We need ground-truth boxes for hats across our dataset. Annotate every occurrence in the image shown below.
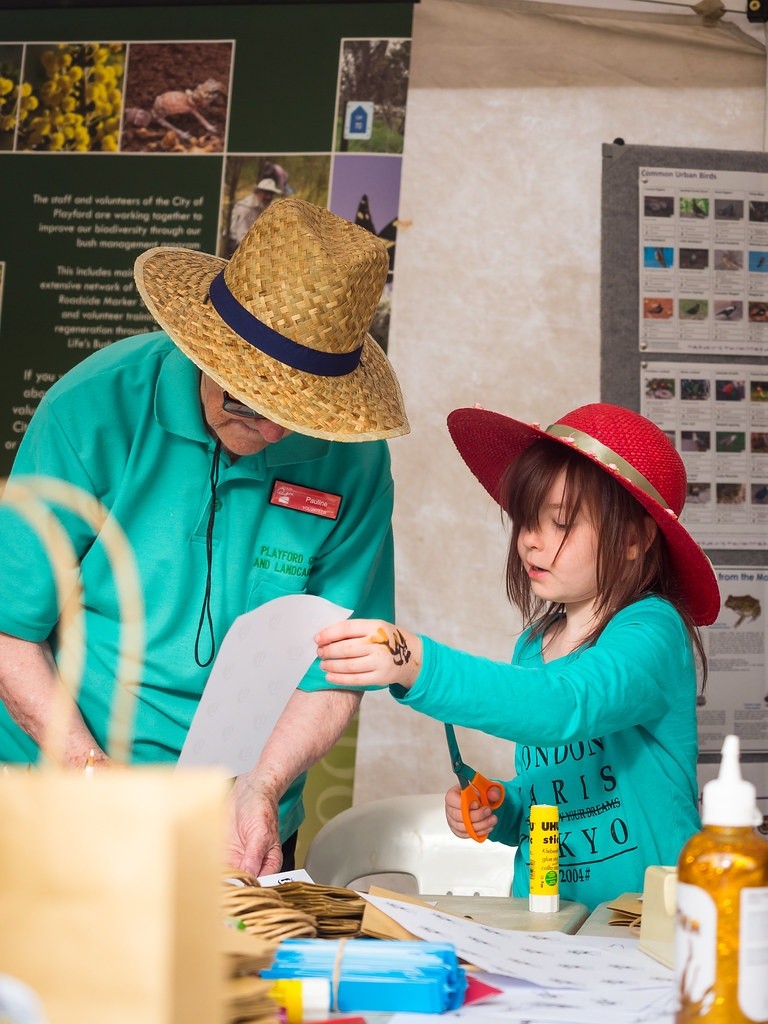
[134,198,411,443]
[447,402,721,627]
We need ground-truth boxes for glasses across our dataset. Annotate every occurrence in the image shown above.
[222,389,267,419]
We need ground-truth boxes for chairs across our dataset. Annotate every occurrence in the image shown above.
[307,793,516,896]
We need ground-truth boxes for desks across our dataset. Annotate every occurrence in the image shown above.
[423,893,640,938]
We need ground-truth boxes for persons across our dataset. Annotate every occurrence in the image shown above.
[315,403,722,913]
[222,177,281,260]
[0,197,411,878]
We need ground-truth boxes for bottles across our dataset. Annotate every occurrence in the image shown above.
[675,736,768,1024]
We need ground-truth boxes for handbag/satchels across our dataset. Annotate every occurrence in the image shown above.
[0,474,235,1024]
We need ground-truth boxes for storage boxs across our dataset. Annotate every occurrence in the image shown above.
[261,936,469,1014]
[637,864,676,971]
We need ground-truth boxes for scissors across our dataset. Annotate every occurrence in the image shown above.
[444,723,505,844]
